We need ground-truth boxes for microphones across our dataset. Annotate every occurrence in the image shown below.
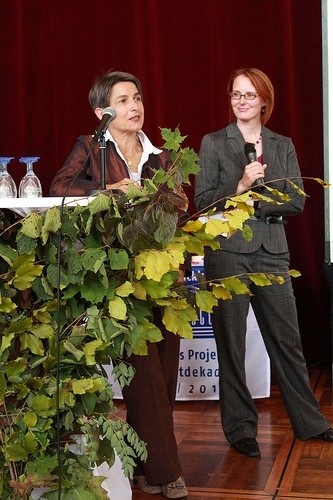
[91,107,116,143]
[244,143,265,191]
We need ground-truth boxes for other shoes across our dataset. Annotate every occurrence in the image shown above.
[130,475,163,495]
[162,474,188,498]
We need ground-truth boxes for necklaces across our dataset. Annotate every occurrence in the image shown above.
[253,133,262,145]
[122,147,139,168]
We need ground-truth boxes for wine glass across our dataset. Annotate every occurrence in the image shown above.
[0,157,17,199]
[18,157,42,198]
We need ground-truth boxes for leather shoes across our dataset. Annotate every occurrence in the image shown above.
[230,438,261,457]
[313,426,333,442]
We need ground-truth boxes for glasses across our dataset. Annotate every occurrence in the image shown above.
[228,91,260,100]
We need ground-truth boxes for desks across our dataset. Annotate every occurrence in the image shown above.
[98,257,271,400]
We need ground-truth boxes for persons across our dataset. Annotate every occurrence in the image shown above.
[193,68,333,455]
[50,71,193,498]
